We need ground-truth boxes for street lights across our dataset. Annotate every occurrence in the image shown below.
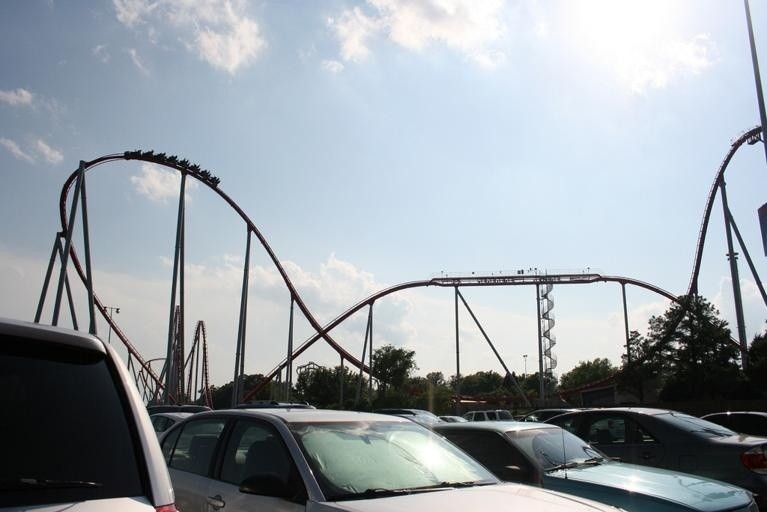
[103,306,120,345]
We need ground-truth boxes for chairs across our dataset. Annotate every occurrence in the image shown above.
[241,440,290,490]
[596,429,619,443]
[187,435,222,477]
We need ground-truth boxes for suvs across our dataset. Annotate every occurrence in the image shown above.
[462,409,514,421]
[259,400,316,409]
[0,316,178,512]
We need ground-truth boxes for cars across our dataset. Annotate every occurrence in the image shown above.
[539,407,767,494]
[700,412,767,437]
[518,408,579,423]
[156,407,627,512]
[146,403,226,446]
[374,408,442,427]
[439,414,469,422]
[426,420,761,512]
